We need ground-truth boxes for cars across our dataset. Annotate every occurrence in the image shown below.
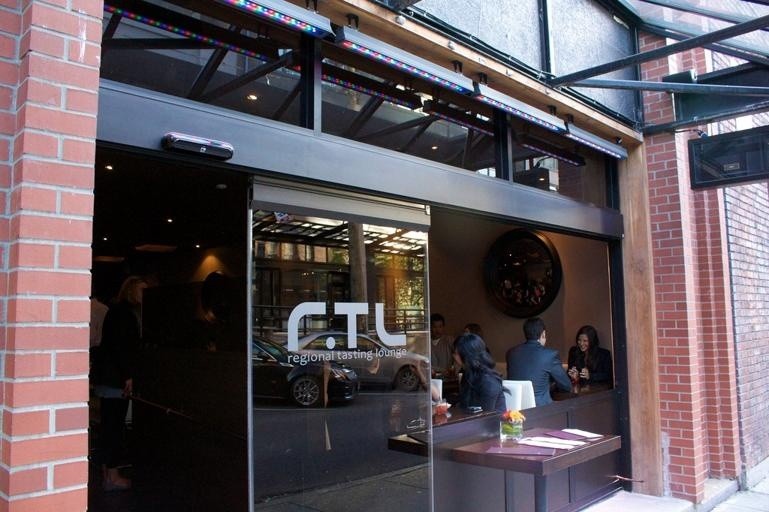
[282,330,431,392]
[252,335,361,407]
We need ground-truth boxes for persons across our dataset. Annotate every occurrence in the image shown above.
[408,358,441,402]
[464,323,491,355]
[505,317,573,407]
[430,313,456,378]
[451,333,507,413]
[93,274,152,493]
[566,325,612,384]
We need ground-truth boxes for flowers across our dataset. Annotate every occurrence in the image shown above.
[500,409,527,436]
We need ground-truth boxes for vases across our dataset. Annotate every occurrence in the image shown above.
[500,423,522,441]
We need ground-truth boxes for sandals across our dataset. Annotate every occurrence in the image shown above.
[104,477,132,491]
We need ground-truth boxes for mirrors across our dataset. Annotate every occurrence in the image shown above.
[482,229,563,317]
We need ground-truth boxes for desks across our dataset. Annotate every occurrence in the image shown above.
[435,428,623,510]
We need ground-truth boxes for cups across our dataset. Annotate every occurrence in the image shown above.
[431,379,443,405]
[499,419,523,442]
